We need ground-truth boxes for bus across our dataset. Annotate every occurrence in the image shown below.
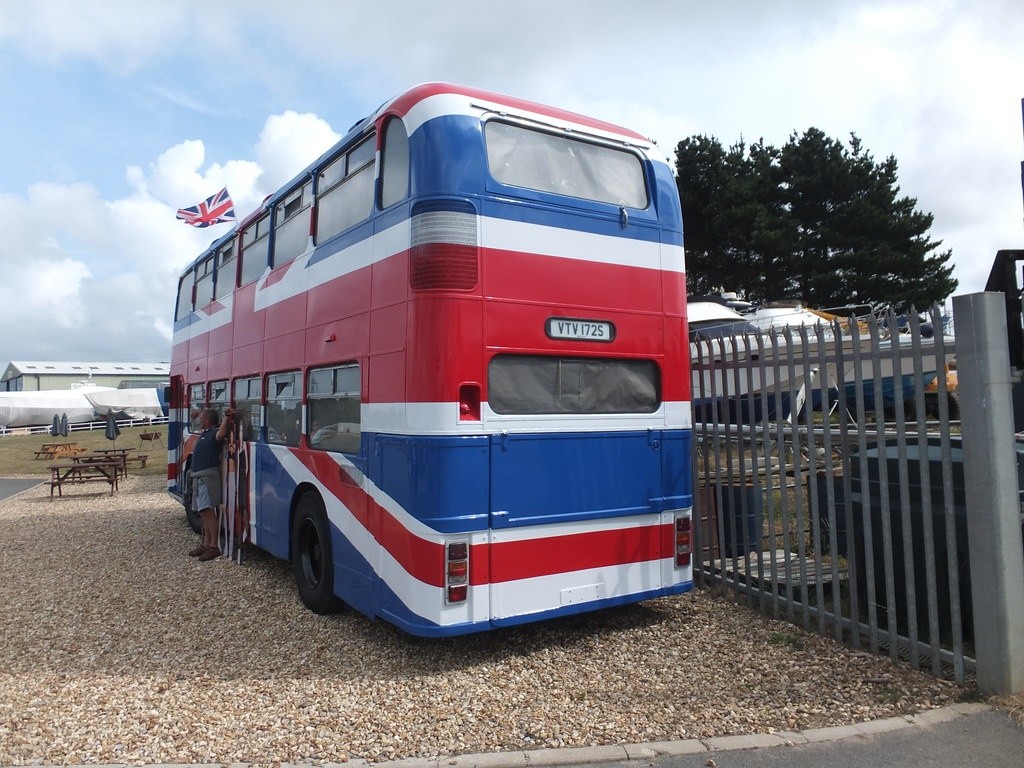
[156,80,696,639]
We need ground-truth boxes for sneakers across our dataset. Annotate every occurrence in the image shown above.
[199,546,221,561]
[189,545,208,556]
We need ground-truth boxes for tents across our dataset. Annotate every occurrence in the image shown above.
[0,387,170,427]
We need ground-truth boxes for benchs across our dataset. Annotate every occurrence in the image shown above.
[44,448,87,456]
[32,450,74,459]
[54,473,124,492]
[67,458,141,481]
[43,478,120,498]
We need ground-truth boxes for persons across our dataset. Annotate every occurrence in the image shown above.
[189,407,233,561]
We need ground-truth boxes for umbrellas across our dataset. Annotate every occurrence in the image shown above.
[52,413,68,443]
[105,408,121,458]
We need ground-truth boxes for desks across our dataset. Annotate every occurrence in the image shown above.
[46,462,122,502]
[35,442,78,459]
[68,453,129,479]
[93,448,137,478]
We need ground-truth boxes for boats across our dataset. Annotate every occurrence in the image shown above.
[684,288,957,421]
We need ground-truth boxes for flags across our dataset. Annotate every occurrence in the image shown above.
[176,186,237,228]
